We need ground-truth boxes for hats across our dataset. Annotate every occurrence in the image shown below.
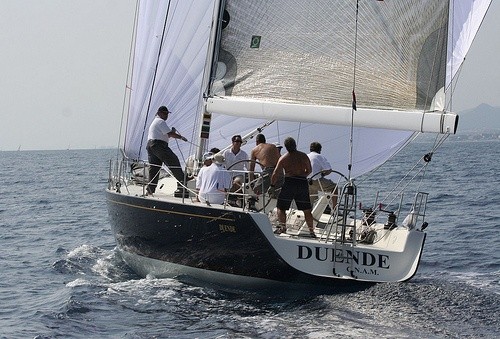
[155,106,171,116]
[274,142,283,147]
[203,152,213,160]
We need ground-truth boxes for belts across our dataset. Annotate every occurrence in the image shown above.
[149,139,166,143]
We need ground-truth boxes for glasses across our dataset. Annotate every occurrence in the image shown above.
[233,140,243,143]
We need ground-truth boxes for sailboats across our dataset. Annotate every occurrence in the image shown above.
[103,1,493,298]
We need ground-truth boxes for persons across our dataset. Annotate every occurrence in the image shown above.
[196,135,338,238]
[146,105,194,196]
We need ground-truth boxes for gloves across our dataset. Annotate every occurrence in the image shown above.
[181,136,187,141]
[172,127,176,133]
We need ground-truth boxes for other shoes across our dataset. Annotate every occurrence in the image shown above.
[310,233,316,237]
[274,228,286,233]
[146,187,153,193]
[184,177,194,181]
[330,210,342,215]
